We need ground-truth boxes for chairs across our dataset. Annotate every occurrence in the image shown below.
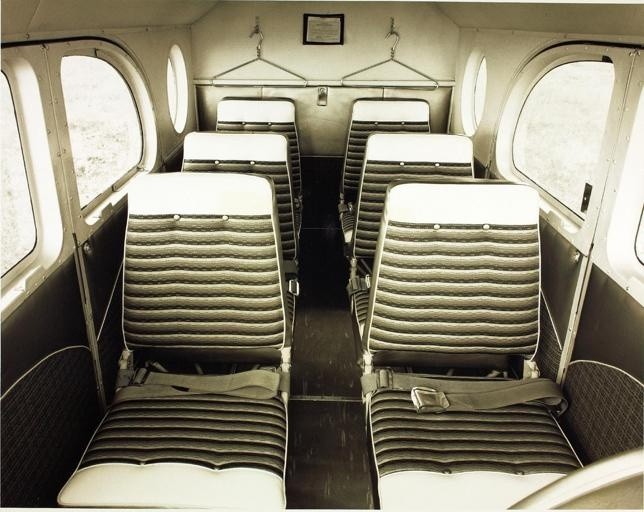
[348,132,475,368]
[215,98,304,235]
[361,180,584,510]
[338,98,431,255]
[56,173,289,509]
[182,131,299,338]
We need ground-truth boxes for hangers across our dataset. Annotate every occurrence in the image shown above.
[340,32,439,92]
[210,31,308,91]
